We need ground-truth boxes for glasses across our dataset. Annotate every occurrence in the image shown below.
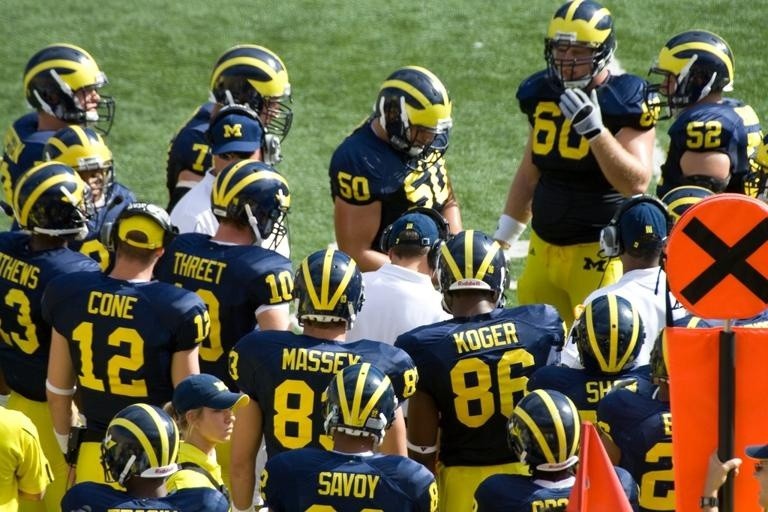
[754,463,768,472]
[216,151,255,160]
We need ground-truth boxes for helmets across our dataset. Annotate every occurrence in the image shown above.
[749,133,768,204]
[650,315,712,386]
[209,160,291,240]
[42,125,115,208]
[324,363,400,446]
[292,249,365,329]
[209,44,293,143]
[23,43,109,126]
[11,161,92,241]
[508,389,581,473]
[100,403,180,487]
[544,0,618,94]
[376,65,453,173]
[649,29,733,107]
[571,295,646,374]
[436,229,510,314]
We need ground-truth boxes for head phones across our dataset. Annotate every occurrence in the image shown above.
[209,104,285,167]
[597,193,676,259]
[380,207,450,269]
[100,201,179,252]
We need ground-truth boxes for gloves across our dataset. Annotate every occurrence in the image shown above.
[559,88,605,143]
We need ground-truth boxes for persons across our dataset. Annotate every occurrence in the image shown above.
[1,160,103,511]
[227,250,418,512]
[492,0,660,331]
[645,30,768,200]
[472,389,640,512]
[343,212,453,345]
[1,374,250,511]
[328,66,461,273]
[525,196,768,512]
[394,230,568,512]
[171,104,292,258]
[260,363,438,512]
[166,44,294,211]
[0,43,134,273]
[41,202,211,489]
[156,160,295,392]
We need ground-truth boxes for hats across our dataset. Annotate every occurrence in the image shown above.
[387,213,439,246]
[172,373,251,418]
[745,444,768,460]
[119,213,165,250]
[618,201,667,249]
[209,111,263,154]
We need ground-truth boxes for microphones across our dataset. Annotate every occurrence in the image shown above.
[96,195,124,242]
